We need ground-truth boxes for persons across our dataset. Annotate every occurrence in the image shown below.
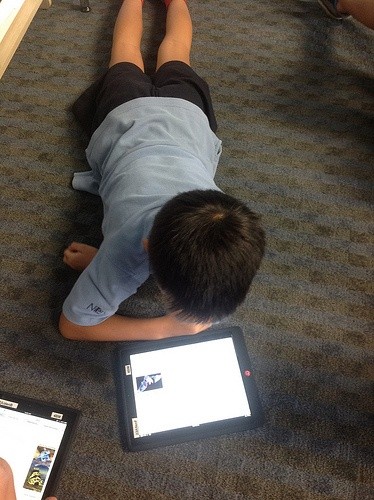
[0,456,58,500]
[318,0,374,31]
[58,0,267,341]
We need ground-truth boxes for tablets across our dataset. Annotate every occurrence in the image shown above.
[114,326,265,453]
[0,391,82,500]
